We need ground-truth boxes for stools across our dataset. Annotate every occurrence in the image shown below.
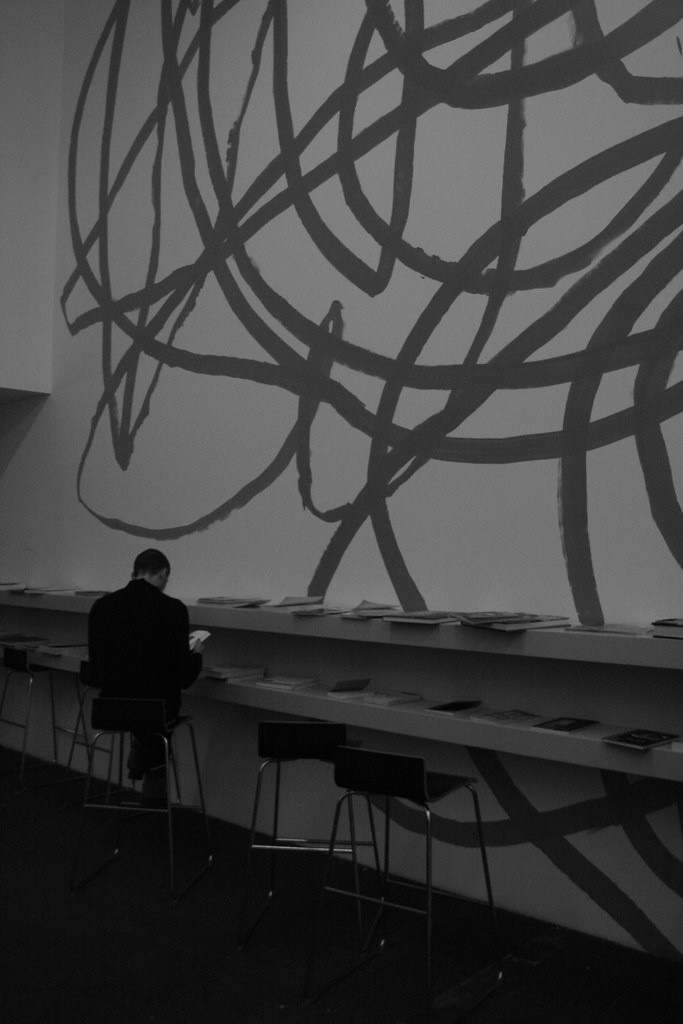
[70,699,211,900]
[301,744,506,1024]
[0,643,93,788]
[232,719,389,950]
[67,658,134,807]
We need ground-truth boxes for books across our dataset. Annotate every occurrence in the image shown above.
[0,583,112,648]
[188,594,682,750]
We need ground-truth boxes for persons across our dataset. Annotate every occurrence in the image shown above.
[88,548,204,780]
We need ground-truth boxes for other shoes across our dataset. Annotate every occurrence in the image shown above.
[128,753,154,781]
